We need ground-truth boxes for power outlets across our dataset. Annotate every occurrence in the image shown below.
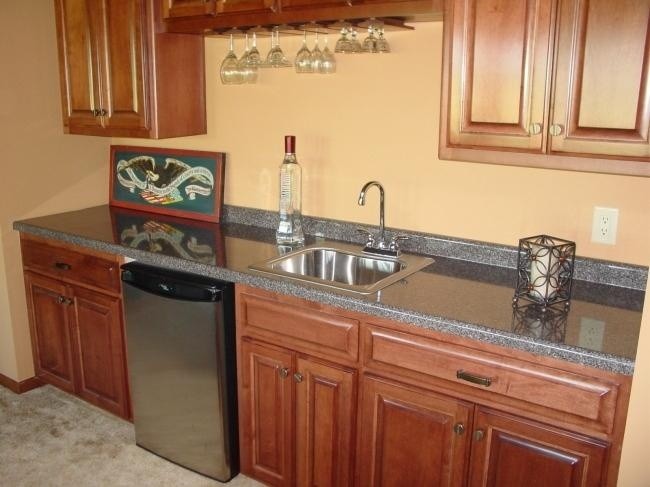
[588,206,618,246]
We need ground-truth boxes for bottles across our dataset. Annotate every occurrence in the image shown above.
[275,134,304,248]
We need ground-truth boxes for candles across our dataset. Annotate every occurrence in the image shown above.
[529,243,561,302]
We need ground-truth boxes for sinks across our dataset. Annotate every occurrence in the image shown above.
[261,247,405,291]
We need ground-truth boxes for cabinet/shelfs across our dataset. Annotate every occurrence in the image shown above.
[16,229,132,426]
[357,315,635,486]
[436,0,650,179]
[234,281,364,486]
[153,0,447,33]
[55,0,207,138]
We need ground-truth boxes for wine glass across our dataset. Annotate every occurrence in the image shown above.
[220,17,391,86]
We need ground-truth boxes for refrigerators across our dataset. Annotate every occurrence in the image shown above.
[119,261,236,484]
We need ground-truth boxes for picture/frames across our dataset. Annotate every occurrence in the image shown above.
[107,144,226,225]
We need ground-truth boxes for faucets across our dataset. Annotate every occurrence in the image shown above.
[358,181,389,250]
